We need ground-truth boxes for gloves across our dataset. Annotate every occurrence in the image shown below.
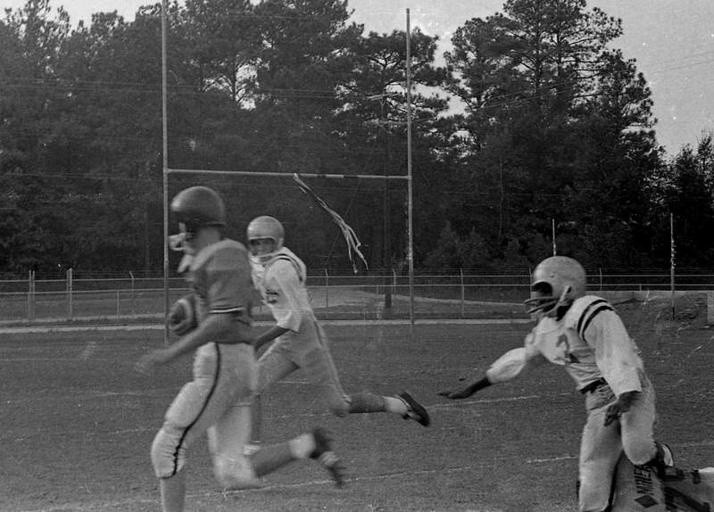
[436,390,469,399]
[604,393,632,426]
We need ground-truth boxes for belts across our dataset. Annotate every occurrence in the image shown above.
[581,377,607,395]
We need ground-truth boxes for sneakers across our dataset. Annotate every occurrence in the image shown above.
[309,428,343,489]
[392,391,429,426]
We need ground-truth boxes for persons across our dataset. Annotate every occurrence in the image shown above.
[436,256,674,511]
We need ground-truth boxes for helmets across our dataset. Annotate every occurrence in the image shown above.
[529,255,586,300]
[247,215,284,254]
[170,186,225,225]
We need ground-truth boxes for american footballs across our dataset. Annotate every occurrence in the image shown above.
[166,293,196,336]
